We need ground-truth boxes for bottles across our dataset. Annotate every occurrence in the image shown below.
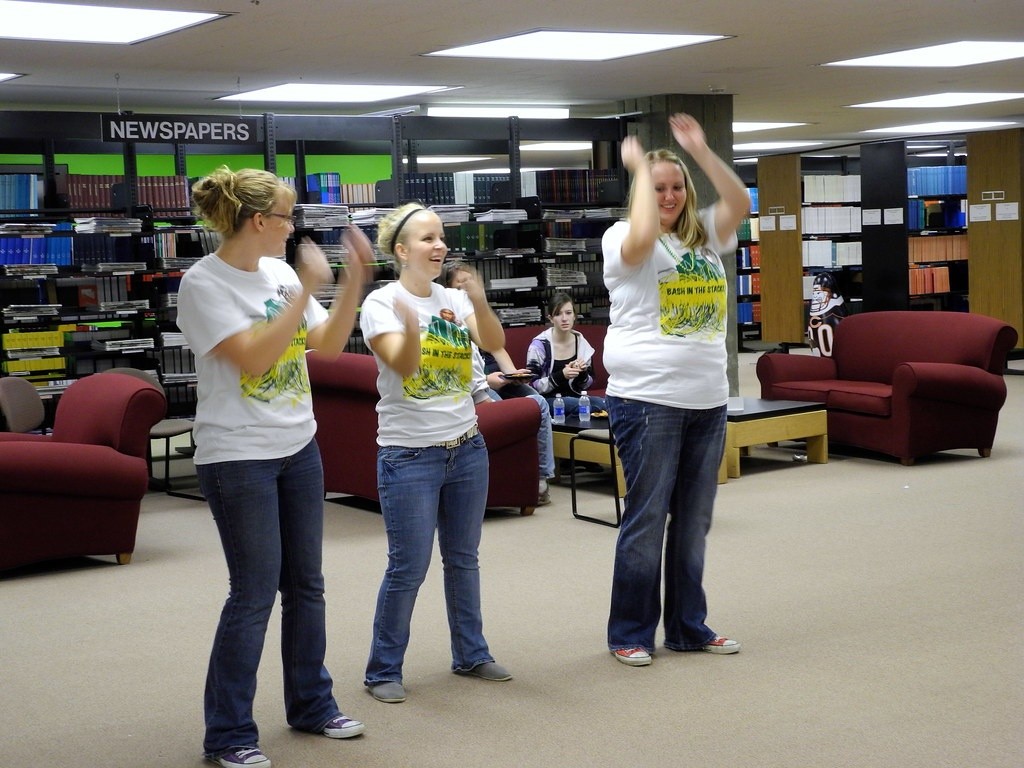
[553,394,565,424]
[578,391,591,421]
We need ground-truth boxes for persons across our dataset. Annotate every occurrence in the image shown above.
[438,261,555,505]
[527,292,606,470]
[603,115,751,667]
[175,168,372,768]
[360,204,512,702]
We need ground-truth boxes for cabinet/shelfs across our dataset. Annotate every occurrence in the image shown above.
[0,113,631,411]
[861,139,968,314]
[761,153,863,351]
[736,165,764,344]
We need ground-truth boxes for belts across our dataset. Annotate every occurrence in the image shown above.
[430,424,478,450]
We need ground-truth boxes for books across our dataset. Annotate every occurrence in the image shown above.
[908,166,969,295]
[801,174,862,300]
[737,217,760,323]
[282,168,625,354]
[0,170,223,403]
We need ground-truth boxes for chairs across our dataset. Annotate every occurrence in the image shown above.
[0,376,44,432]
[109,368,203,492]
[570,421,622,531]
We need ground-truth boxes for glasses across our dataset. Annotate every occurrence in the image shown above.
[250,211,296,225]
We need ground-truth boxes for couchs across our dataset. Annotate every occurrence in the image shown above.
[491,324,616,404]
[303,347,539,514]
[755,311,1019,465]
[0,374,166,565]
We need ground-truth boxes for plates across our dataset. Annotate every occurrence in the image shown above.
[591,416,608,420]
[504,373,539,378]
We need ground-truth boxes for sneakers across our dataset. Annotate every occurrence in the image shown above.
[611,646,652,666]
[323,714,365,739]
[702,636,741,654]
[204,748,271,768]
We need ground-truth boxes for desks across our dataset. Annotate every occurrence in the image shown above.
[722,397,828,478]
[549,413,728,496]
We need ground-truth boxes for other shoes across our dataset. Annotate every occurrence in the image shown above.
[367,681,407,703]
[537,481,551,506]
[452,661,513,681]
[559,464,572,476]
[585,462,604,473]
